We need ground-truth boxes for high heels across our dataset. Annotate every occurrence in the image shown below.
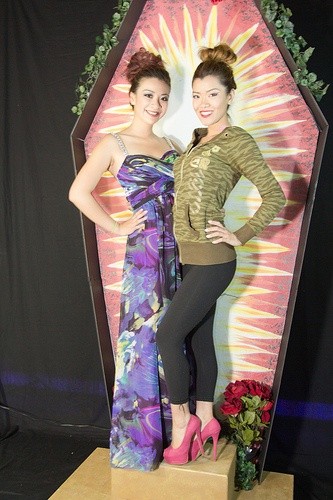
[188,418,221,461]
[163,413,205,464]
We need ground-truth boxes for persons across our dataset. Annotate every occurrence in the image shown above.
[67,47,186,472]
[154,41,287,467]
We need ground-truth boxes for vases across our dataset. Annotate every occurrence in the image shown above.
[236,444,259,489]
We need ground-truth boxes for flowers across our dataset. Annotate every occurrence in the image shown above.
[220,380,273,490]
